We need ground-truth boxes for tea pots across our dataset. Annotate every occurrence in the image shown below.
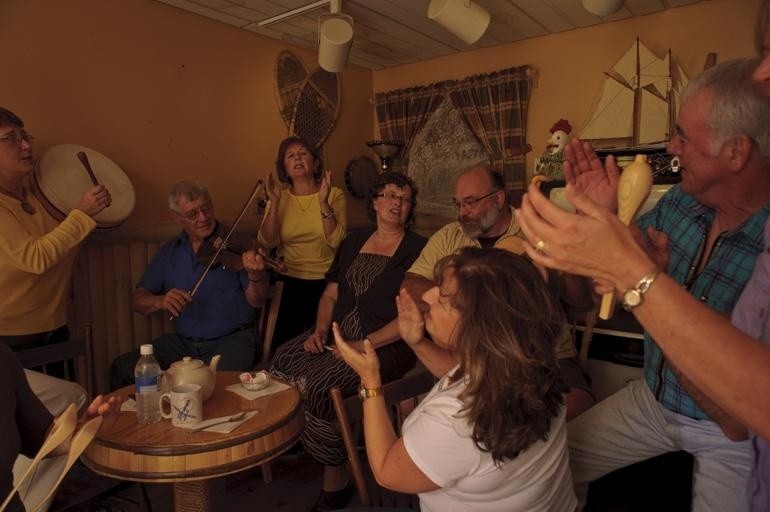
[157,353,221,402]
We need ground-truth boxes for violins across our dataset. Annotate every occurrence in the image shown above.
[189,220,289,281]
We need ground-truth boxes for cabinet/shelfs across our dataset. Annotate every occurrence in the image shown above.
[574,324,643,404]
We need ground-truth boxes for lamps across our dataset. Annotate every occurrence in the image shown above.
[367,140,405,173]
[316,13,354,74]
[582,0,627,16]
[426,0,491,45]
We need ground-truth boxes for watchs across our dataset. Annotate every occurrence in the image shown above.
[620,264,664,313]
[358,385,386,401]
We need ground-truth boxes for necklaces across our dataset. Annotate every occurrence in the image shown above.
[0,184,37,216]
[293,185,318,213]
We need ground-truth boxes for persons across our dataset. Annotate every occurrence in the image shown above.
[108,171,270,400]
[513,0,770,512]
[271,170,429,511]
[561,56,770,511]
[0,106,113,512]
[256,133,348,365]
[0,342,123,511]
[394,162,596,424]
[331,245,579,512]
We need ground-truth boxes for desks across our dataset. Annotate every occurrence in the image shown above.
[78,369,307,512]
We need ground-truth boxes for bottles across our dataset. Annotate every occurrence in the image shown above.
[134,343,163,425]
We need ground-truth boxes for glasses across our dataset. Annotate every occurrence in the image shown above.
[0,134,35,144]
[375,193,410,202]
[453,191,497,211]
[177,205,211,221]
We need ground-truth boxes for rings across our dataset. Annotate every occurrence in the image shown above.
[534,239,547,252]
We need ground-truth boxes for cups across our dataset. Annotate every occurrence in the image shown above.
[159,384,203,431]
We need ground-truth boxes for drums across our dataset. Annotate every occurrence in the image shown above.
[29,141,138,237]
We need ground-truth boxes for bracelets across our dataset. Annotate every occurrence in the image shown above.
[320,206,335,220]
[247,274,267,282]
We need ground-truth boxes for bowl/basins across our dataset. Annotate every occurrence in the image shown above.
[242,376,269,391]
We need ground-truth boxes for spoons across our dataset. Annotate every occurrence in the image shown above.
[187,410,246,435]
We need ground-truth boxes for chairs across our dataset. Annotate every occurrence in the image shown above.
[331,367,441,512]
[8,324,150,512]
[254,279,285,485]
